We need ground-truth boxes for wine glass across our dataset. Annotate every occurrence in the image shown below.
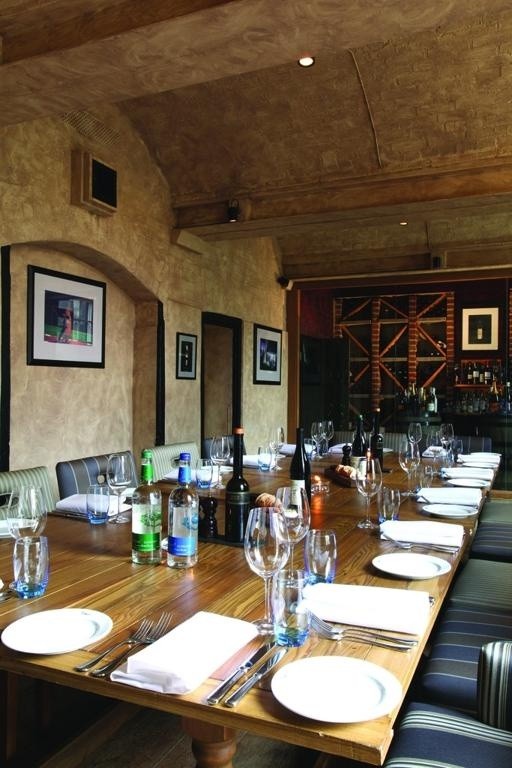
[211,432,230,488]
[311,423,324,459]
[425,430,442,477]
[271,427,284,471]
[106,453,133,524]
[399,441,422,497]
[6,486,50,594]
[354,458,383,529]
[408,423,423,471]
[321,420,334,456]
[241,487,311,638]
[439,423,452,450]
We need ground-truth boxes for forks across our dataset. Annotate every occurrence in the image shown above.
[305,611,419,654]
[73,611,174,679]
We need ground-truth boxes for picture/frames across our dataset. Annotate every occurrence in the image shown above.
[455,299,506,359]
[26,263,107,367]
[252,322,284,385]
[175,330,198,380]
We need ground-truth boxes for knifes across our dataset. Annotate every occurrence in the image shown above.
[208,638,292,706]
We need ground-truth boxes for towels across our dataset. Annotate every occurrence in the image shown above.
[457,454,500,464]
[164,468,211,480]
[379,520,465,554]
[230,454,270,465]
[55,493,126,514]
[328,442,352,452]
[416,488,482,506]
[109,610,263,694]
[440,468,495,481]
[279,443,316,455]
[294,583,431,638]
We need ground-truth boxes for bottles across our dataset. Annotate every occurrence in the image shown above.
[395,359,511,419]
[350,415,368,477]
[166,453,200,569]
[288,428,311,512]
[130,448,163,566]
[225,428,250,542]
[370,412,384,472]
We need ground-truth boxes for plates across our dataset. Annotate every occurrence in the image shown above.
[371,451,501,581]
[1,608,114,656]
[271,654,404,728]
[2,519,39,539]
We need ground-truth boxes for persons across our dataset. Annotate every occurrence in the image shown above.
[57,314,70,340]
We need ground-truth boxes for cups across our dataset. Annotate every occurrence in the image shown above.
[258,447,273,472]
[86,484,111,527]
[452,440,464,463]
[12,536,51,600]
[377,487,400,522]
[304,438,314,458]
[195,458,213,489]
[269,528,340,648]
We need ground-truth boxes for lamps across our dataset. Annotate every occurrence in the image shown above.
[226,198,241,223]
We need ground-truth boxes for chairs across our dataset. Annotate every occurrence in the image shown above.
[331,430,354,444]
[458,435,493,454]
[56,449,139,500]
[0,465,56,520]
[142,441,201,482]
[380,642,512,767]
[202,435,247,467]
[382,432,408,452]
[422,498,512,702]
